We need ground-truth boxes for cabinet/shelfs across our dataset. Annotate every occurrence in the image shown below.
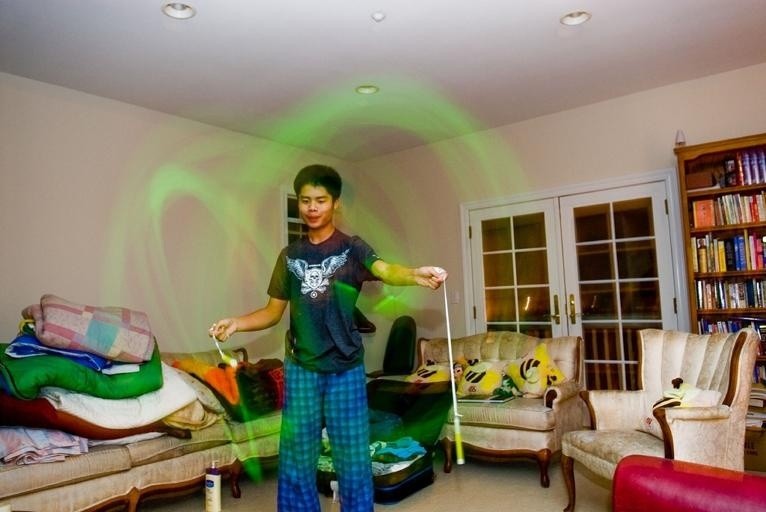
[672,136,765,333]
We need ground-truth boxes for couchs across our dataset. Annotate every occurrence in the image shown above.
[378,329,596,487]
[0,345,284,511]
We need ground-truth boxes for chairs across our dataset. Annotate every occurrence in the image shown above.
[557,327,761,512]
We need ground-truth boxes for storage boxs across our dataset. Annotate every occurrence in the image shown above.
[744,425,766,471]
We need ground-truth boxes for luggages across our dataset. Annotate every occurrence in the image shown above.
[316,379,458,504]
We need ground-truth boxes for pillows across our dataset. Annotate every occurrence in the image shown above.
[400,340,569,400]
[634,379,722,443]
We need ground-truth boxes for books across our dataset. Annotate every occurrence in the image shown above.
[457,395,516,404]
[690,148,765,430]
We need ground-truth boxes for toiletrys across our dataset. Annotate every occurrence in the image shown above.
[206,460,222,512]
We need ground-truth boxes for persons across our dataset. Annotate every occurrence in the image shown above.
[207,164,449,512]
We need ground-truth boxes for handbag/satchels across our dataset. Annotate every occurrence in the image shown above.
[202,348,284,423]
